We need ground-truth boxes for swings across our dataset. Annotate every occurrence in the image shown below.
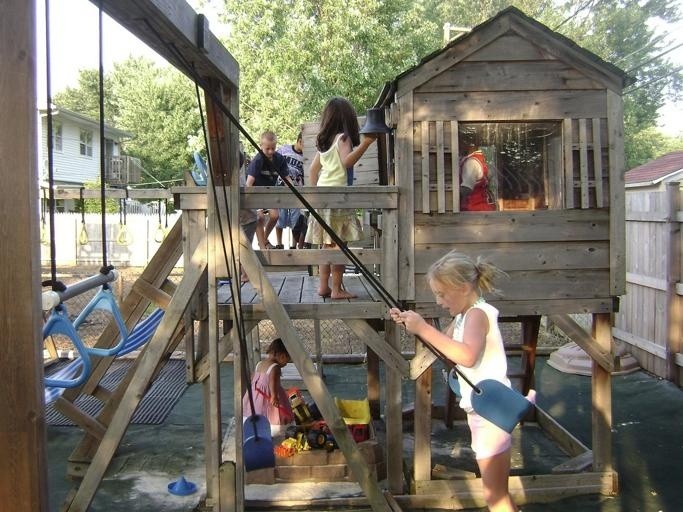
[201,84,530,434]
[201,94,278,471]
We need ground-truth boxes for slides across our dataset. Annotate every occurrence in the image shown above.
[44,307,165,403]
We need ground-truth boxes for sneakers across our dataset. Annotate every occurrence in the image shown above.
[258,240,275,249]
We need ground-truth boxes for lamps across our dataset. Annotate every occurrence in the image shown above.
[359,107,392,137]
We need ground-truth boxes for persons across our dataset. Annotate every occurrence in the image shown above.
[275,130,302,249]
[246,132,294,251]
[238,151,258,281]
[390,249,526,512]
[458,130,497,211]
[241,337,296,425]
[310,96,377,299]
